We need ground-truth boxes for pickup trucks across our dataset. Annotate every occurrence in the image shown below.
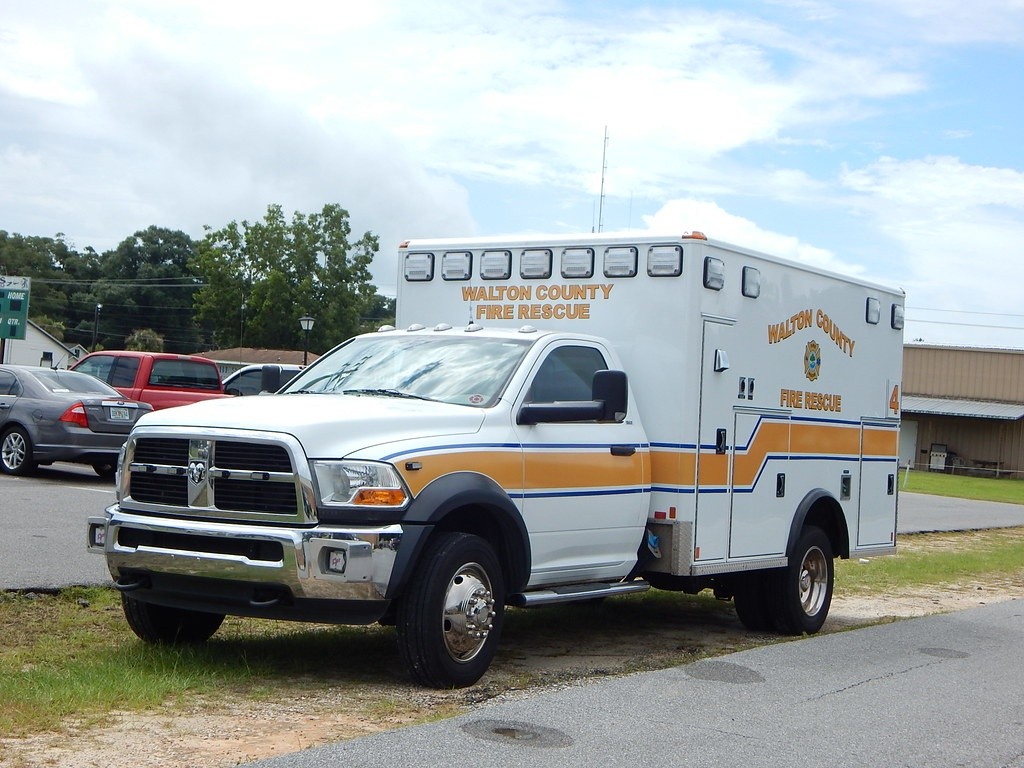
[68,350,237,412]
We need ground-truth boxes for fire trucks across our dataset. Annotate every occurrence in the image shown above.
[86,232,910,692]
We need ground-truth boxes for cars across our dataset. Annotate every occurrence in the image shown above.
[0,362,155,481]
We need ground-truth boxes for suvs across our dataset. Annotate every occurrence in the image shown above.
[222,364,312,398]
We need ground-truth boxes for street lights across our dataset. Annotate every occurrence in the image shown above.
[297,311,318,367]
[92,303,104,353]
